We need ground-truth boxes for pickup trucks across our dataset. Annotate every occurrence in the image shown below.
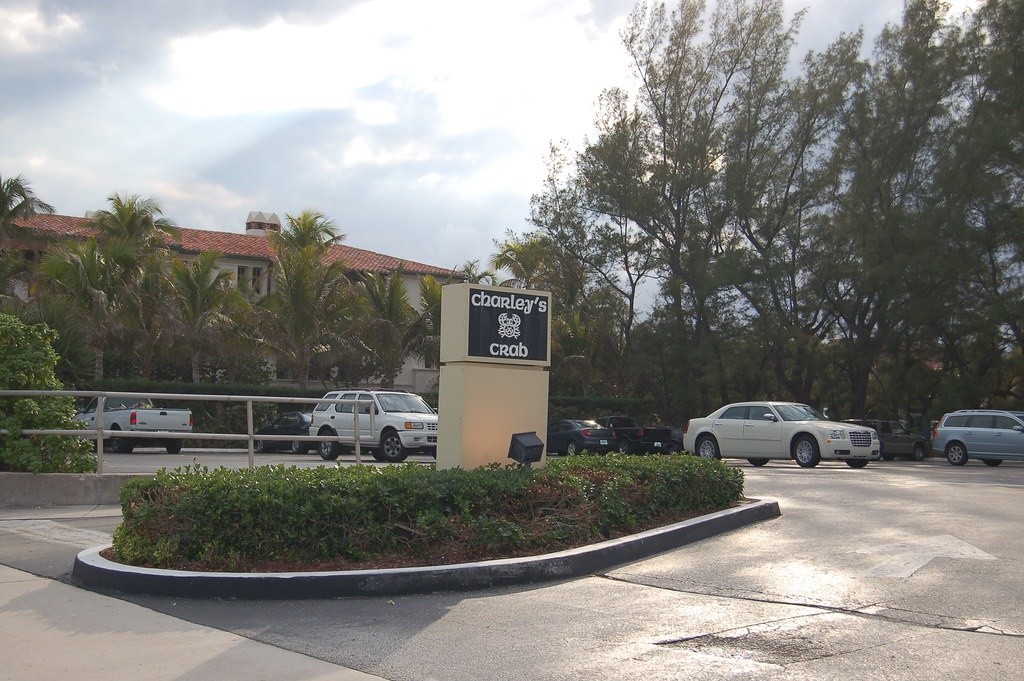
[595,415,673,456]
[71,395,194,456]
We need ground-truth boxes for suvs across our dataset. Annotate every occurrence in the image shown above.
[308,386,438,464]
[931,408,1024,467]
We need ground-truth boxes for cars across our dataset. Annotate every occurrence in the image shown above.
[840,417,928,461]
[547,419,618,456]
[643,425,685,455]
[683,400,881,469]
[253,408,318,455]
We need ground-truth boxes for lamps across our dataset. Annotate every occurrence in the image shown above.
[507,429,545,469]
